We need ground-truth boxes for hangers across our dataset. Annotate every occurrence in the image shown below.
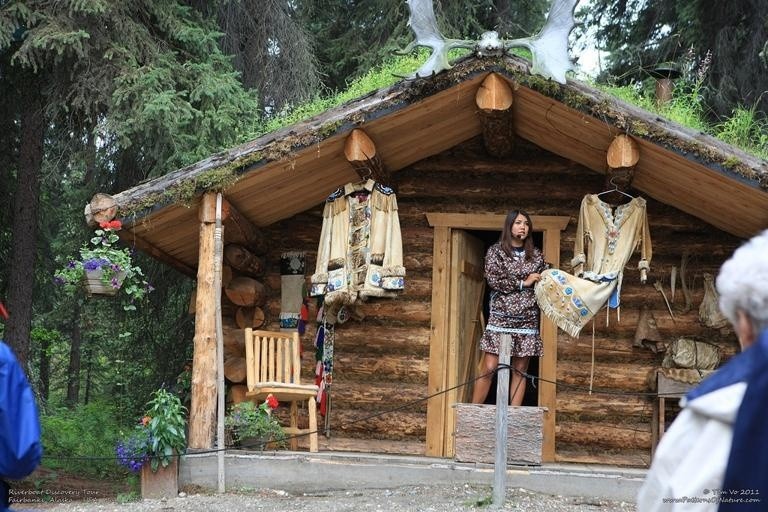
[596,174,635,210]
[352,166,372,187]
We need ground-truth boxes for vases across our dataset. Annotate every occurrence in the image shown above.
[240,431,270,451]
[82,266,127,298]
[140,448,180,500]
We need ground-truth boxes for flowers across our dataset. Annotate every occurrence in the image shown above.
[55,219,155,311]
[226,392,287,451]
[116,380,189,474]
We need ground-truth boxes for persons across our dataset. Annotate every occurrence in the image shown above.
[635,228,768,512]
[469,210,552,406]
[1,305,43,512]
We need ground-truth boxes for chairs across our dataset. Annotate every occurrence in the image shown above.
[242,326,321,452]
